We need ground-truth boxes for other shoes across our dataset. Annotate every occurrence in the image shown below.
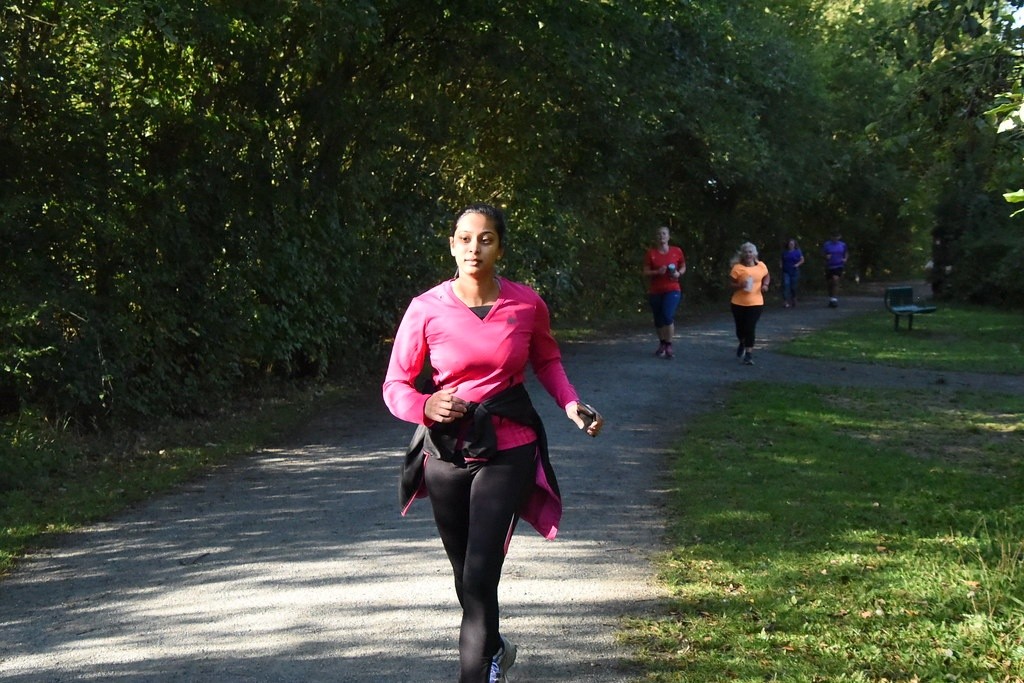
[737,343,745,357]
[657,341,666,357]
[829,299,838,308]
[743,353,753,364]
[665,342,675,358]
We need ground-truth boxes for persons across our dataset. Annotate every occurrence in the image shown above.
[641,226,687,361]
[383,207,605,683]
[727,243,771,367]
[778,239,805,308]
[820,230,849,308]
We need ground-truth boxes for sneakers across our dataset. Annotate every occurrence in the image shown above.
[490,636,517,683]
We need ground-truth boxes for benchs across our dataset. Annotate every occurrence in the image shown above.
[884,285,938,333]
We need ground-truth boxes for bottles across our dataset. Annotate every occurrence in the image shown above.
[744,275,753,292]
[668,264,675,278]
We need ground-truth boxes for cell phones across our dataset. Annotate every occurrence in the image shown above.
[576,401,596,438]
